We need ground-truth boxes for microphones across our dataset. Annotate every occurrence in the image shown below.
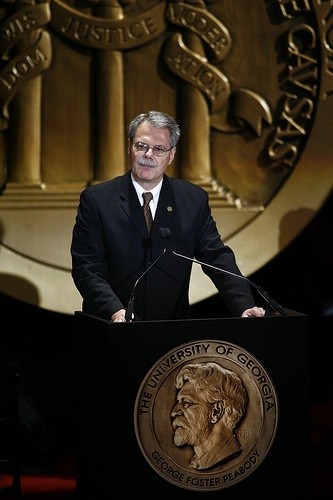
[125,228,167,322]
[166,228,307,317]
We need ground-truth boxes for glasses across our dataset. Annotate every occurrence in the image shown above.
[134,142,173,157]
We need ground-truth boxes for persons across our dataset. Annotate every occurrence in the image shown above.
[170,362,248,471]
[70,111,265,322]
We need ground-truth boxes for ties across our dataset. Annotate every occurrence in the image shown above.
[142,193,153,237]
